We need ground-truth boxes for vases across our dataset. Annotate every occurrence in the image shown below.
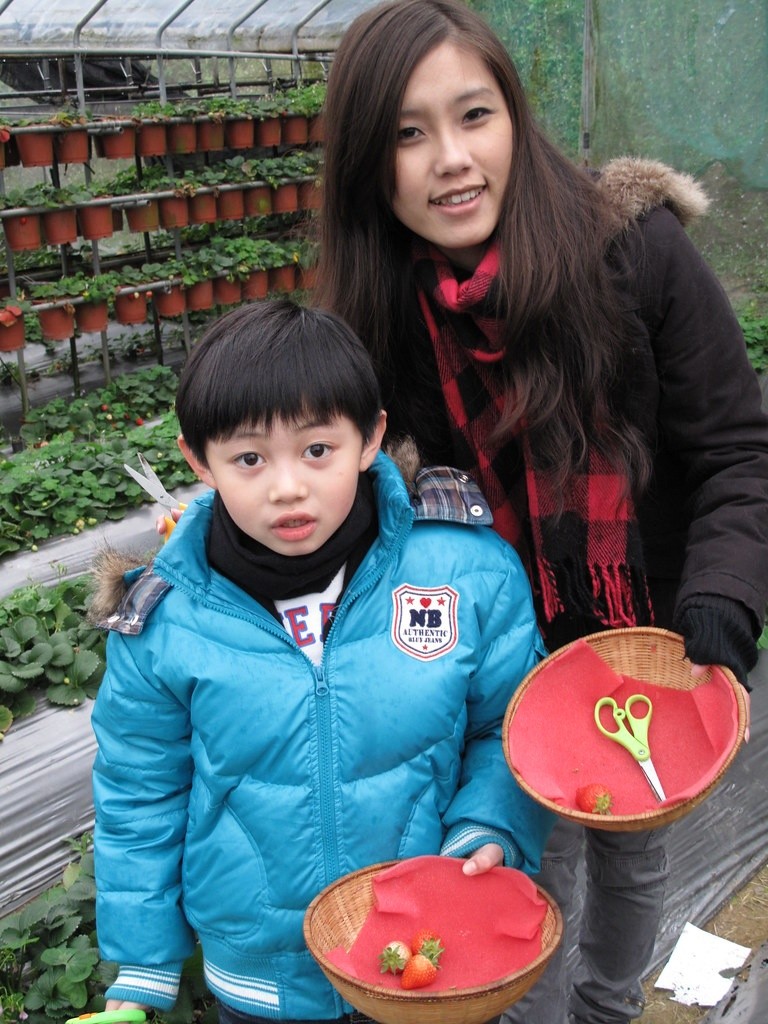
[0,235,317,352]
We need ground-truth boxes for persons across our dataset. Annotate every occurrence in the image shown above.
[154,0,768,1023]
[92,301,559,1024]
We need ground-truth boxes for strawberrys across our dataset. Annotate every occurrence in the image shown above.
[576,783,612,814]
[0,404,185,551]
[114,284,153,300]
[379,929,447,990]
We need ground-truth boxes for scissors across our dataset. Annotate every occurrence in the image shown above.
[123,452,189,543]
[595,693,666,802]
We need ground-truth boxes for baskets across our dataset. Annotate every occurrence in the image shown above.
[502,625,745,832]
[303,858,564,1024]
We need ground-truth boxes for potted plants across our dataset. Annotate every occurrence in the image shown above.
[117,166,158,232]
[242,157,271,217]
[295,148,325,210]
[259,156,301,214]
[186,167,217,225]
[39,182,77,245]
[158,176,188,229]
[0,181,39,251]
[77,183,114,240]
[218,155,250,219]
[0,79,328,167]
